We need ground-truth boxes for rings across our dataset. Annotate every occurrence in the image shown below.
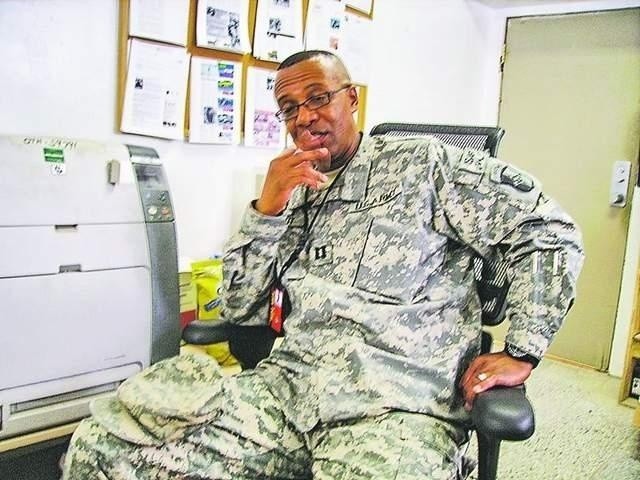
[477,372,488,381]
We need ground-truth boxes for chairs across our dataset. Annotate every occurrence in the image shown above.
[182,123,535,480]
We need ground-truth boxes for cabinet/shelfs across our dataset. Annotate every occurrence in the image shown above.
[618,253,640,409]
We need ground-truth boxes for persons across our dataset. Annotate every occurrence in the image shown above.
[59,49,584,480]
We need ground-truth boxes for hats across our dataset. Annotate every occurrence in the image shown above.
[89,354,240,446]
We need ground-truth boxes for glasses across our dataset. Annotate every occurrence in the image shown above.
[276,83,354,122]
